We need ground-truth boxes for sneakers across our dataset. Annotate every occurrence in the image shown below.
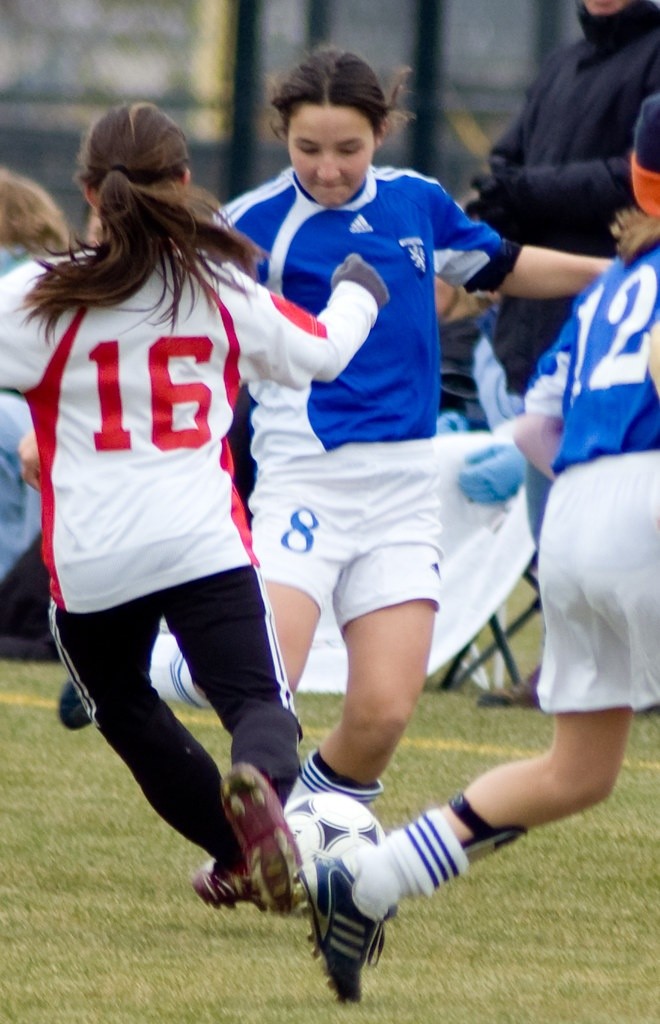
[221,764,305,914]
[194,852,265,911]
[299,846,400,1007]
[61,682,95,729]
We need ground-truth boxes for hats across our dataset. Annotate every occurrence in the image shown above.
[631,96,660,223]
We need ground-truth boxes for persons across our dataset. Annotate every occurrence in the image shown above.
[467,0,660,708]
[437,193,497,418]
[83,202,259,528]
[19,47,616,813]
[0,165,95,639]
[290,97,660,1006]
[0,104,389,916]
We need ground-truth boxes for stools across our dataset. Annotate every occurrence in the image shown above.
[438,553,541,691]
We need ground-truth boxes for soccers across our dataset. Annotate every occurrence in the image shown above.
[283,790,392,906]
